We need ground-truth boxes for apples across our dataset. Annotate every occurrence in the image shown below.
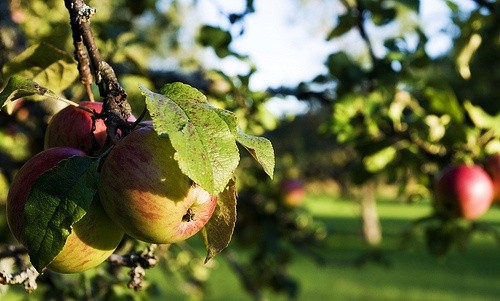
[6,101,217,274]
[279,179,306,206]
[439,152,499,219]
